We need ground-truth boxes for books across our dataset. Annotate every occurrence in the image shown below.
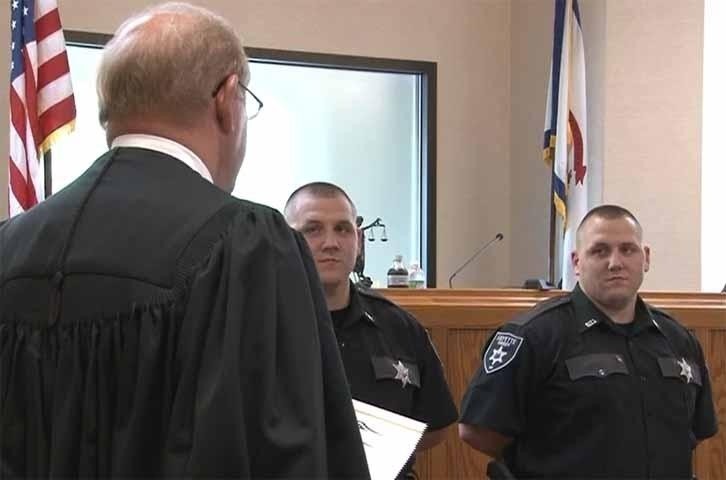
[350,398,428,480]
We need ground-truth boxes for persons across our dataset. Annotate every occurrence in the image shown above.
[281,181,458,480]
[0,2,371,479]
[456,204,718,479]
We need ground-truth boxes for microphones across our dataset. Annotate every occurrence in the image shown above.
[448,233,503,288]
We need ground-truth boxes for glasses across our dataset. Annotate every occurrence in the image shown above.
[210,66,264,124]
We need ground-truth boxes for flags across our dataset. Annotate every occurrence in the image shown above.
[5,1,76,220]
[541,0,586,293]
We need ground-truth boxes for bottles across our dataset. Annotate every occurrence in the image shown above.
[407,264,426,289]
[386,253,408,288]
